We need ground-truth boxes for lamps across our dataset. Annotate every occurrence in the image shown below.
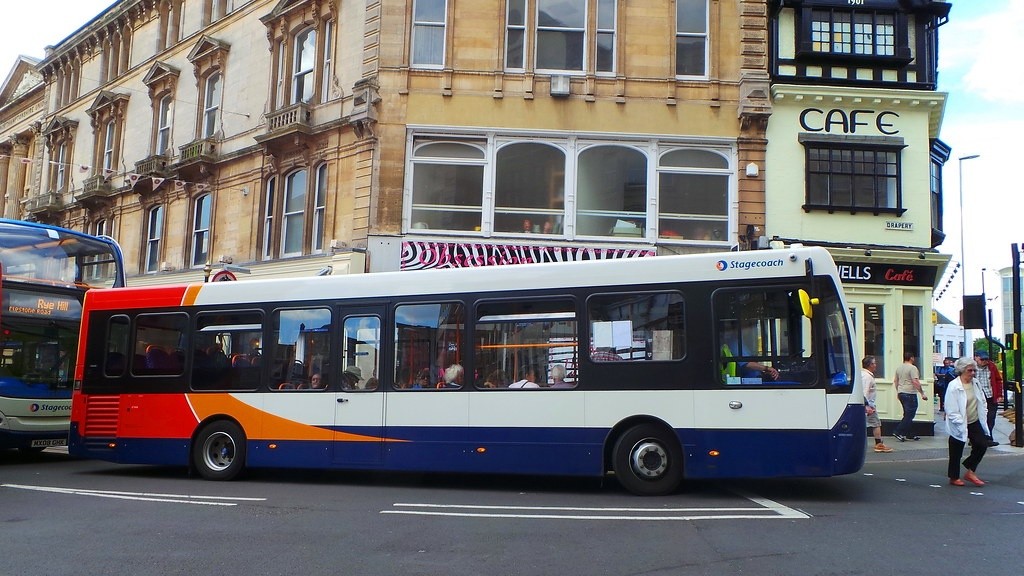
[550,74,571,96]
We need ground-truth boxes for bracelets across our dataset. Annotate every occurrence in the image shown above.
[763,366,768,374]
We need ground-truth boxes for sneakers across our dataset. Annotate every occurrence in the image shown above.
[906,436,920,441]
[874,443,892,451]
[893,432,905,442]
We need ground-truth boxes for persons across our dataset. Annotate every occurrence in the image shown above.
[311,365,379,390]
[5,351,23,375]
[861,355,893,453]
[550,365,574,388]
[508,365,540,388]
[397,364,509,389]
[728,315,779,383]
[892,352,928,442]
[968,350,1003,448]
[935,359,959,420]
[590,337,623,362]
[944,358,991,486]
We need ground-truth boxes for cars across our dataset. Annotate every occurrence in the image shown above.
[997,390,1015,409]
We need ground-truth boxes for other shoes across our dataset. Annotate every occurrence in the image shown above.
[964,472,985,486]
[950,479,964,486]
[987,439,999,446]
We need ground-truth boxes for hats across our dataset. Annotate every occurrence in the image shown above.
[345,366,364,380]
[976,350,989,358]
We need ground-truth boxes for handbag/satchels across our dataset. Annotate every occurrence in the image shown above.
[936,381,945,389]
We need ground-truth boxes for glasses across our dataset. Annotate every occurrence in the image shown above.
[421,376,430,379]
[966,369,977,373]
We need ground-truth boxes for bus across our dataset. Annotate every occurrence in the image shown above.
[0,218,126,453]
[68,240,868,498]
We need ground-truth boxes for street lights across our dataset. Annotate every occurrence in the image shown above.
[982,267,987,294]
[958,152,981,295]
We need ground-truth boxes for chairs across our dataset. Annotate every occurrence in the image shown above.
[105,344,304,389]
